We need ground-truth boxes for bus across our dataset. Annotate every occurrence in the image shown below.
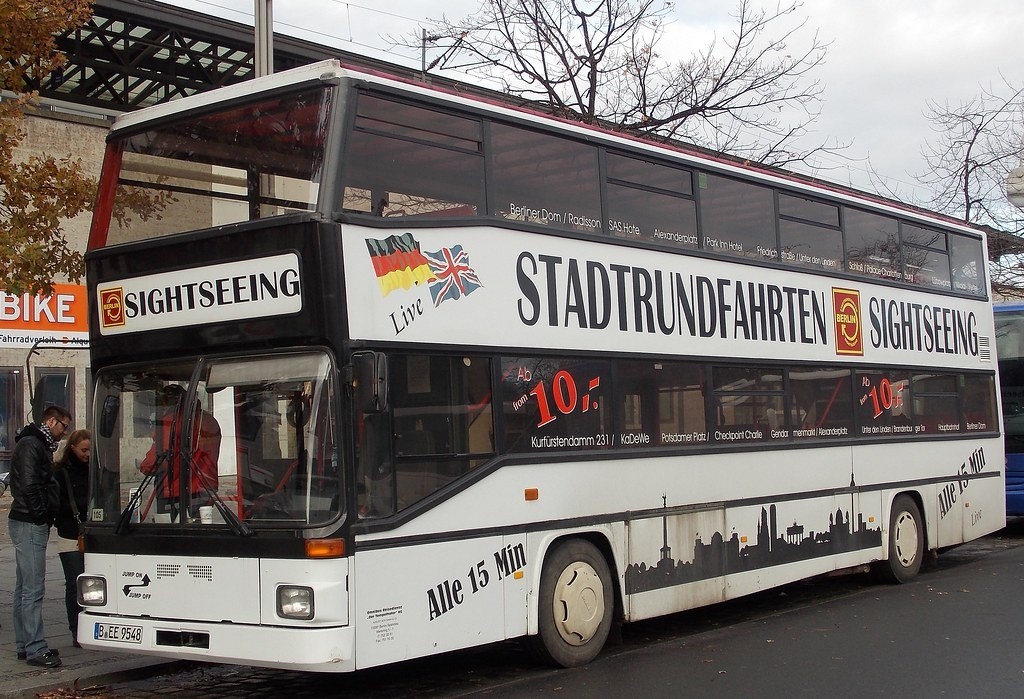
[26,57,1007,676]
[993,304,1024,520]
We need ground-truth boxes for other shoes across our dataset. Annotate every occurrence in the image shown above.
[73,633,82,648]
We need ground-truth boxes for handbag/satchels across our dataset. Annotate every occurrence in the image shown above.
[77,522,86,553]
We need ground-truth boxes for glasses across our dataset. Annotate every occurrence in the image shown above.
[54,418,69,429]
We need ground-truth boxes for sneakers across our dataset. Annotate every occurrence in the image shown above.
[18,648,58,659]
[26,653,62,668]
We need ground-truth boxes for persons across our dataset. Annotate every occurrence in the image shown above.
[357,386,408,486]
[960,383,985,431]
[55,429,91,648]
[8,405,72,667]
[140,384,221,523]
